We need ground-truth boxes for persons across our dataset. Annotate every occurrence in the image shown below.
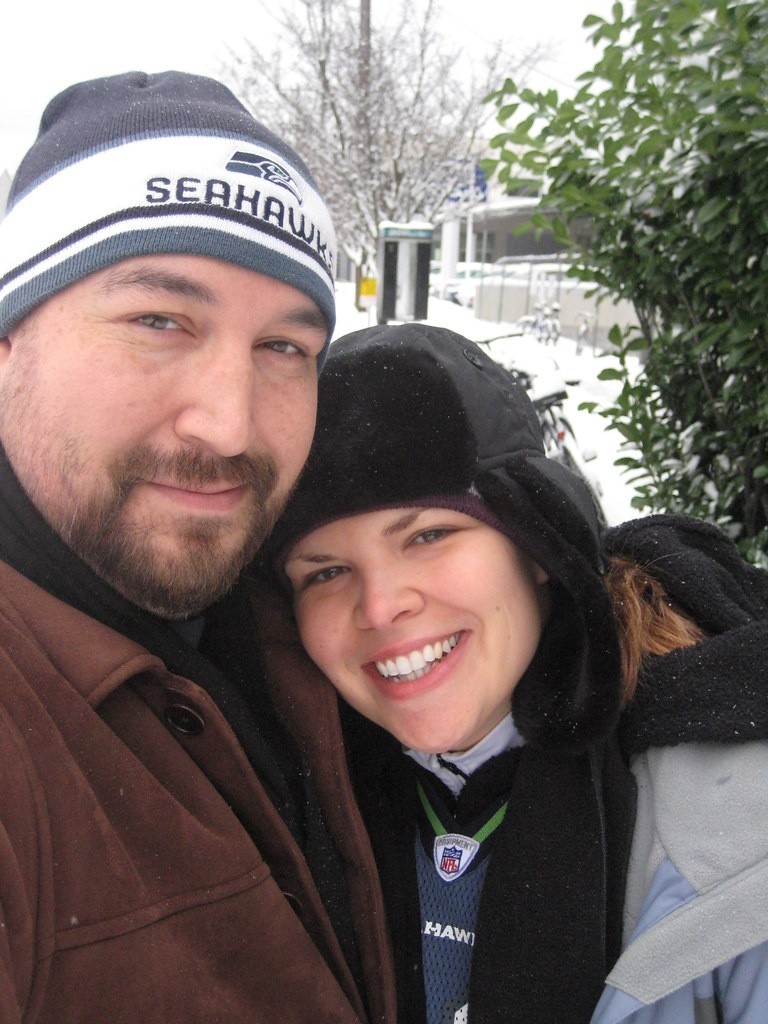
[0,71,396,1024]
[257,321,768,1024]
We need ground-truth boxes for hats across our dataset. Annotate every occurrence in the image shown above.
[251,322,600,603]
[0,69,336,378]
